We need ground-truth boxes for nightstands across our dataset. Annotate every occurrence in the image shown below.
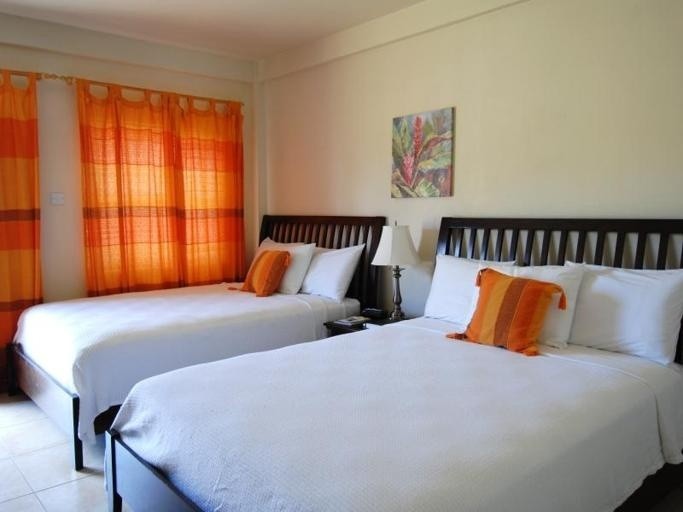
[323,311,416,338]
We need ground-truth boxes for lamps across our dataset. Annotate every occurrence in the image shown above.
[371,219,422,321]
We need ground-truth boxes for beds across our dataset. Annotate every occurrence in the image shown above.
[7,215,387,470]
[104,217,682,512]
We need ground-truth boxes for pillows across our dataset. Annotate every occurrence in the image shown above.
[240,234,366,305]
[423,252,683,367]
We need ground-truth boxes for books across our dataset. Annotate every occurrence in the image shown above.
[333,315,369,327]
